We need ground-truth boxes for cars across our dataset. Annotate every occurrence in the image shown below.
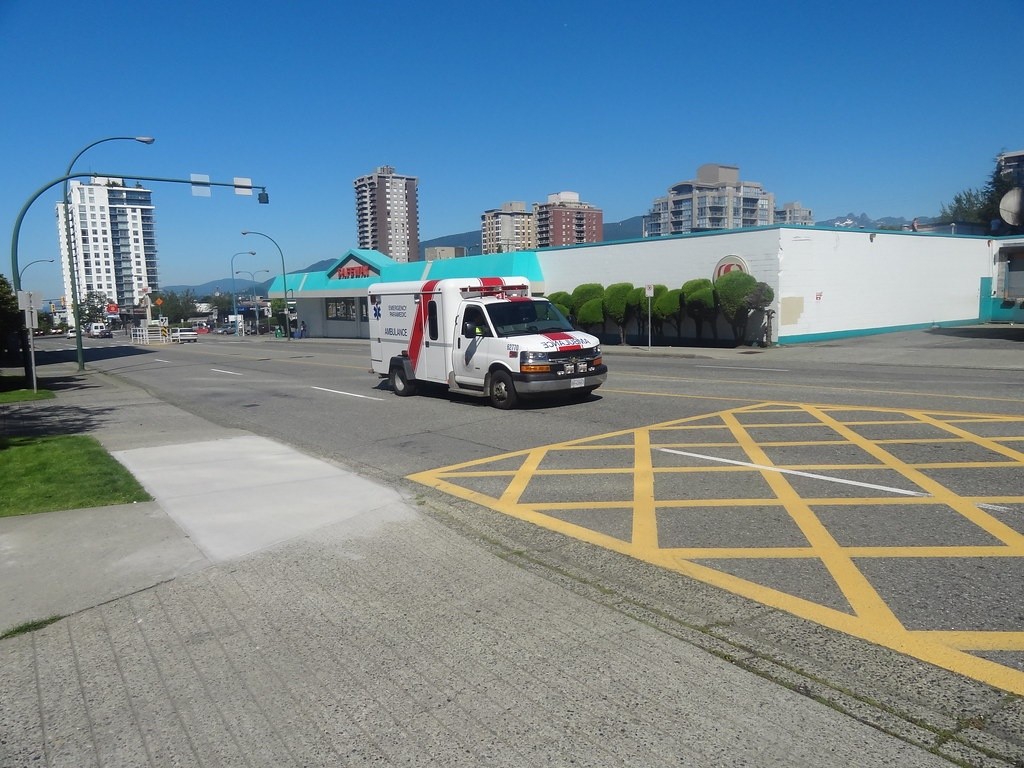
[215,324,235,335]
[66,329,77,339]
[98,330,113,339]
[172,328,198,342]
[255,324,276,332]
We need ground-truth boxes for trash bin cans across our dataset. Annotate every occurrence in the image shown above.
[275,328,282,339]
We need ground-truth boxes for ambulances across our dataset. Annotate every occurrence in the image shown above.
[367,278,608,410]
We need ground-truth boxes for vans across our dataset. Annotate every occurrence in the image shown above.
[90,323,105,338]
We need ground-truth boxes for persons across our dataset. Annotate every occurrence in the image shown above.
[300,320,307,339]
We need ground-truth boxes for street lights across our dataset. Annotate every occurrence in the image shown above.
[231,251,256,336]
[234,270,269,336]
[63,137,156,372]
[20,260,55,279]
[241,231,290,341]
